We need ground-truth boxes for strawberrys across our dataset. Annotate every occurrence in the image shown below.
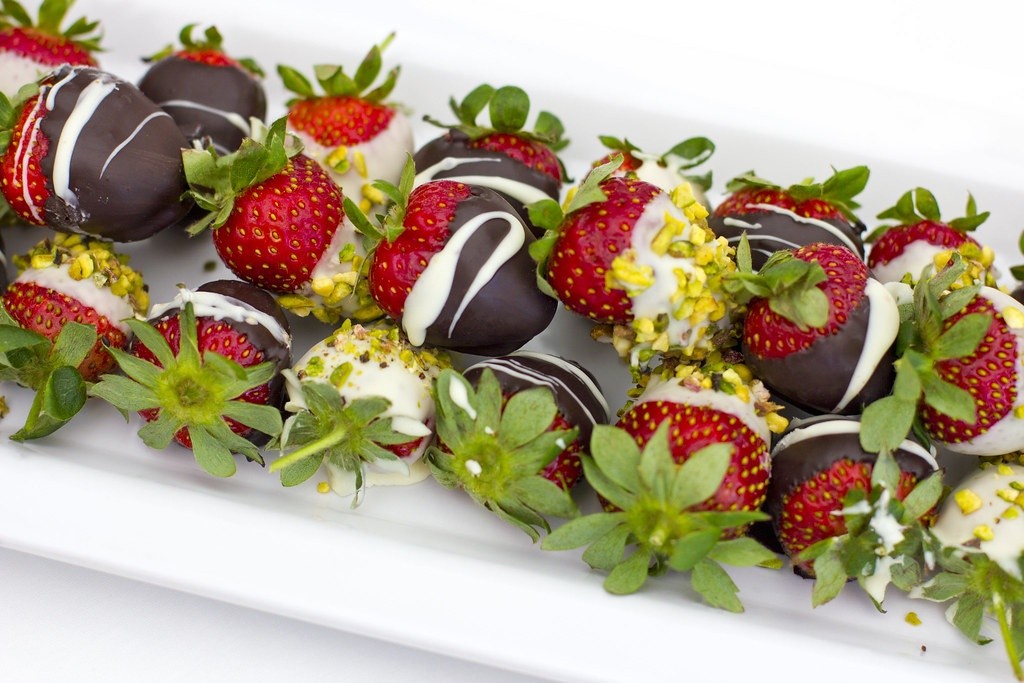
[0,0,1024,682]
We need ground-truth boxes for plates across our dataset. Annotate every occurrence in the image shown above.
[0,0,1024,683]
